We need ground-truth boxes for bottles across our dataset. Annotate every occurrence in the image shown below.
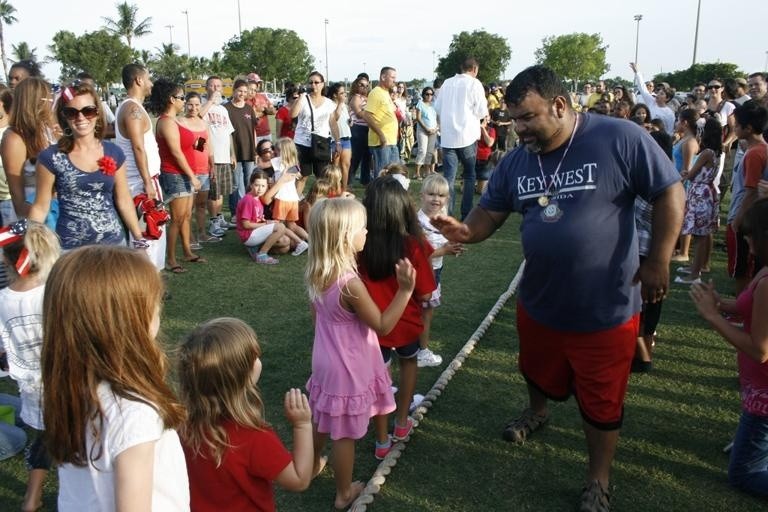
[214,91,223,105]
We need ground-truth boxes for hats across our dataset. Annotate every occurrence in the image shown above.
[246,72,264,84]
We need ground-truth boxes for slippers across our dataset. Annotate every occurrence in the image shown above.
[163,262,189,275]
[190,241,205,250]
[182,254,209,263]
[198,234,224,244]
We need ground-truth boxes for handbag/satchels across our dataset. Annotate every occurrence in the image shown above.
[310,132,332,162]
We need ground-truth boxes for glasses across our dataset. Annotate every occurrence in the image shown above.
[308,81,322,85]
[260,145,275,155]
[707,85,723,89]
[61,105,99,122]
[173,95,185,101]
[424,93,433,96]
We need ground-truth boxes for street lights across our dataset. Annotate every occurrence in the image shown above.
[432,49,436,81]
[342,76,349,96]
[632,12,646,83]
[160,24,177,44]
[181,9,191,54]
[322,16,333,83]
[362,61,367,72]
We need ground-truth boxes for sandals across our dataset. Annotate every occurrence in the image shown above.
[500,408,550,444]
[575,478,613,512]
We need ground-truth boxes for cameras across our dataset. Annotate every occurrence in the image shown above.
[298,86,314,94]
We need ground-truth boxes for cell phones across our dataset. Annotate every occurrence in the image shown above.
[287,166,301,174]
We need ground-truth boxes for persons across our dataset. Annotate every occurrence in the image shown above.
[0,56,512,512]
[686,199,768,498]
[570,62,768,374]
[430,65,686,511]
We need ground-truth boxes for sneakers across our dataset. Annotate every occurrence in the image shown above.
[208,218,226,237]
[392,413,414,442]
[409,392,425,411]
[391,384,398,394]
[161,291,174,301]
[290,241,309,257]
[629,359,654,374]
[216,214,237,231]
[416,349,442,368]
[246,245,280,265]
[372,434,393,459]
[412,166,443,181]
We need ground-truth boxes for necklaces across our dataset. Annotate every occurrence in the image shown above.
[537,111,578,207]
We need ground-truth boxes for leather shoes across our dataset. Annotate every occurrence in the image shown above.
[667,275,702,285]
[676,265,701,277]
